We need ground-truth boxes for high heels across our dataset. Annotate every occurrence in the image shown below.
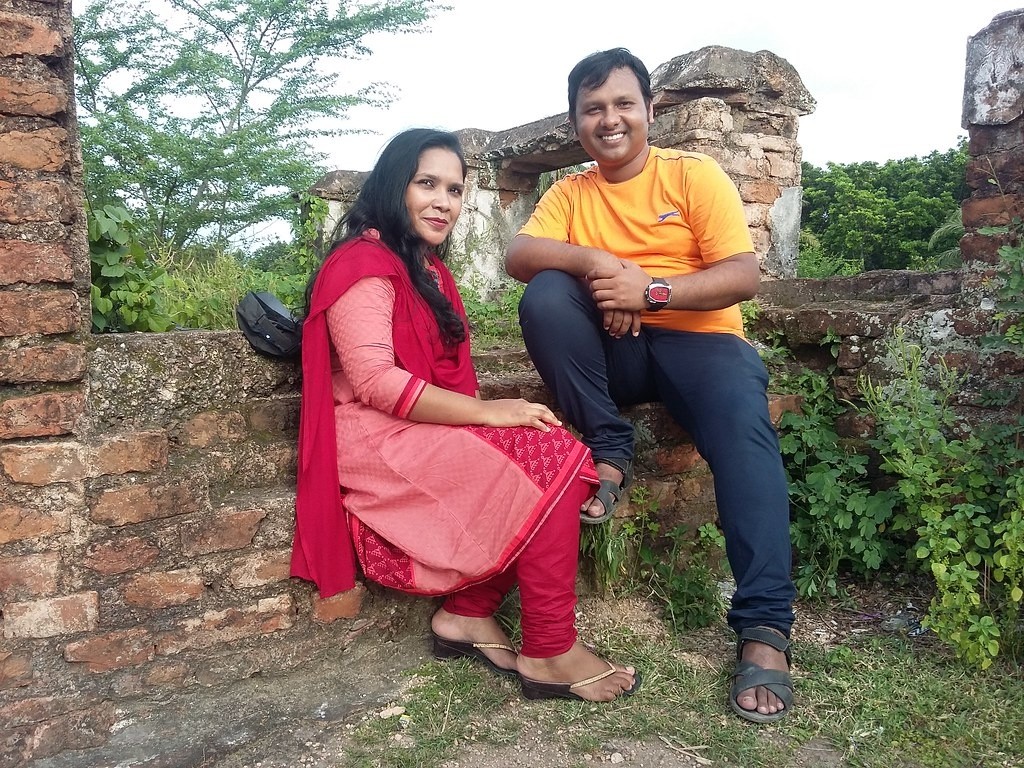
[434,633,519,676]
[519,660,642,703]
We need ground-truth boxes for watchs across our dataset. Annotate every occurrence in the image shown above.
[644,276,672,313]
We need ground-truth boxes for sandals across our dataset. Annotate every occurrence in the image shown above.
[580,457,633,524]
[730,625,794,723]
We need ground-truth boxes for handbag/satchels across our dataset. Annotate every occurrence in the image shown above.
[237,292,303,357]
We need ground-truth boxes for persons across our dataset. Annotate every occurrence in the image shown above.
[503,47,798,724]
[288,127,643,702]
